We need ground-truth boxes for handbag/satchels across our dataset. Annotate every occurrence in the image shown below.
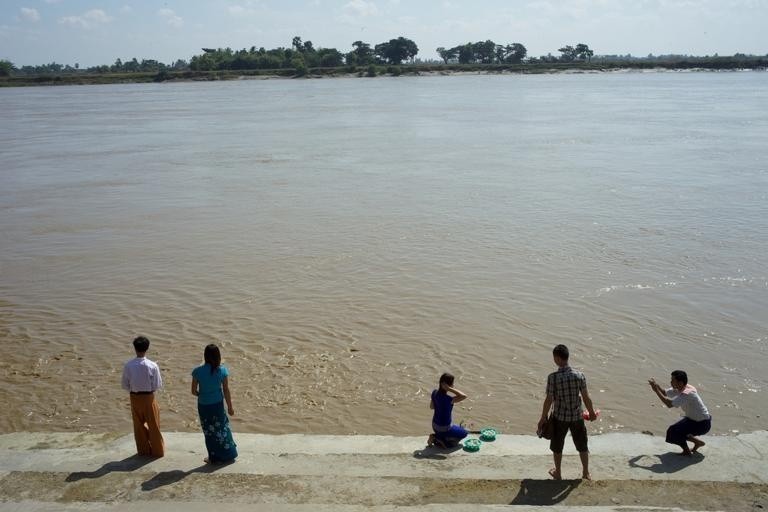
[541,415,557,439]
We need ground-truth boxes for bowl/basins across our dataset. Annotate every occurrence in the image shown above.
[464,428,496,450]
[582,409,600,420]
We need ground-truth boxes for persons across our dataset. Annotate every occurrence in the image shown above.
[535,343,598,481]
[120,337,166,459]
[426,373,468,450]
[192,344,239,467]
[647,370,712,457]
